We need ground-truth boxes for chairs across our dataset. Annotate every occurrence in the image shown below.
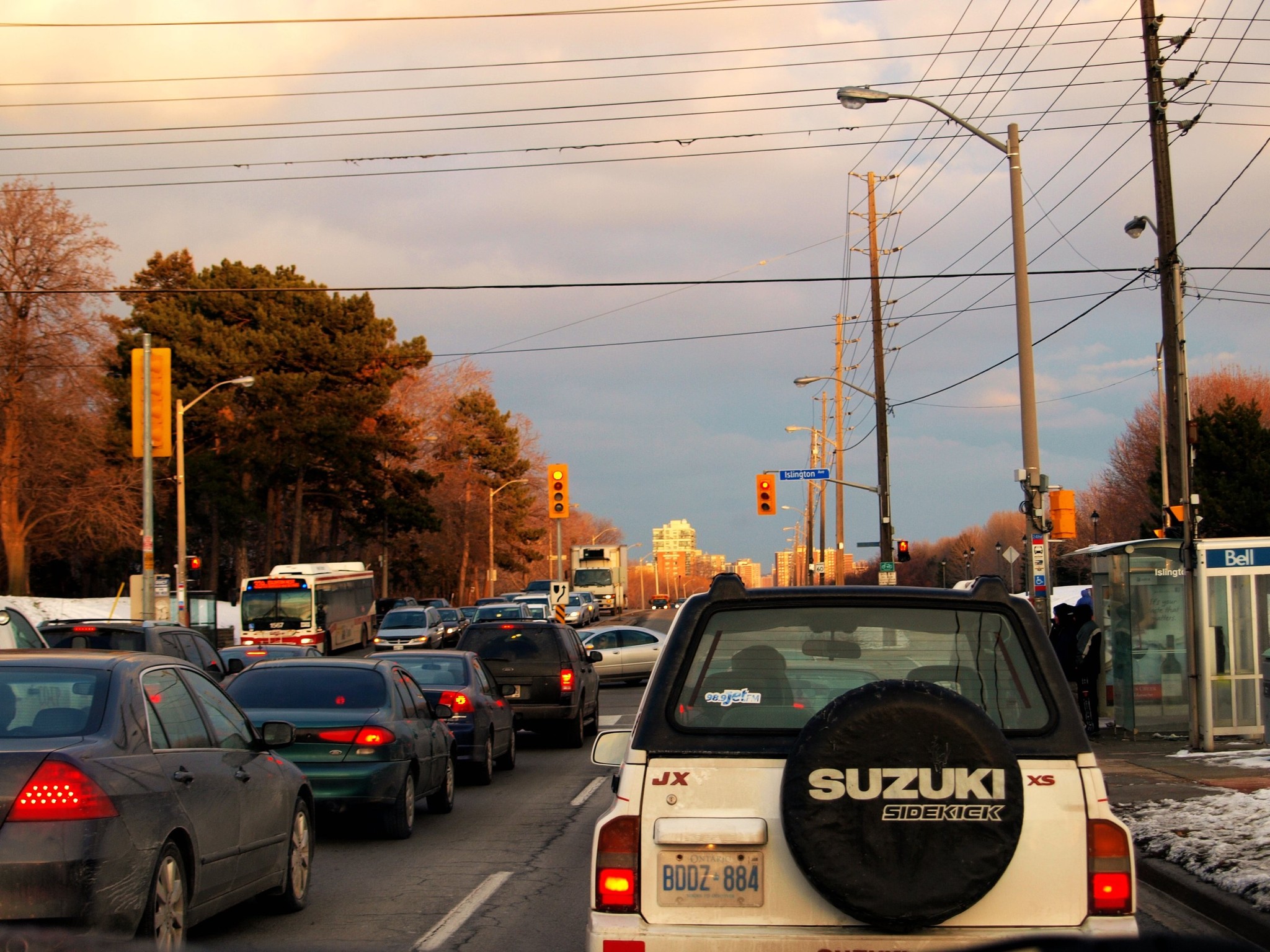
[607,635,616,648]
[905,665,988,709]
[434,671,455,686]
[702,671,786,708]
[33,708,88,734]
[717,703,815,730]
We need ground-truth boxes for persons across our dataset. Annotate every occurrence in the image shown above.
[1047,602,1079,694]
[1073,605,1105,741]
[1075,589,1094,620]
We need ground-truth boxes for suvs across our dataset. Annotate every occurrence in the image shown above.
[590,570,1144,951]
[32,617,236,680]
[460,620,601,744]
[376,606,444,651]
[375,595,417,627]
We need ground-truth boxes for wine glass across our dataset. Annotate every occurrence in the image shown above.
[1132,628,1150,685]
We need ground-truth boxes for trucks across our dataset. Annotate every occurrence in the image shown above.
[565,544,629,615]
[649,594,671,609]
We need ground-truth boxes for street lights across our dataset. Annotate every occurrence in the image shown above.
[628,543,704,612]
[485,478,530,599]
[559,502,581,582]
[784,527,812,587]
[995,539,1003,579]
[788,424,844,586]
[1020,533,1031,596]
[778,502,814,586]
[835,86,1054,617]
[796,373,894,578]
[1129,215,1194,540]
[592,527,618,546]
[1089,509,1101,547]
[173,372,255,632]
[963,545,975,582]
[764,471,827,584]
[942,558,946,591]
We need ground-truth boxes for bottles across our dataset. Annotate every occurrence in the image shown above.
[1160,635,1183,716]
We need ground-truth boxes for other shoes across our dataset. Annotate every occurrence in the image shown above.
[1085,728,1101,738]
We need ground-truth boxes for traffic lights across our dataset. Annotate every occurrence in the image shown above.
[896,541,911,563]
[128,347,173,459]
[548,463,569,520]
[755,473,777,516]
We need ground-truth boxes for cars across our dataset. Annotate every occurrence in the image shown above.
[366,652,524,788]
[438,607,468,644]
[522,578,558,593]
[571,592,601,621]
[2,647,317,945]
[563,594,590,628]
[674,598,687,609]
[226,655,458,842]
[574,628,673,684]
[421,588,554,623]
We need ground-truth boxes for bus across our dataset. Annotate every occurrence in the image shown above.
[244,556,380,656]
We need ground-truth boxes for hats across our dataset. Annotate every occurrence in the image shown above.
[1054,603,1075,614]
